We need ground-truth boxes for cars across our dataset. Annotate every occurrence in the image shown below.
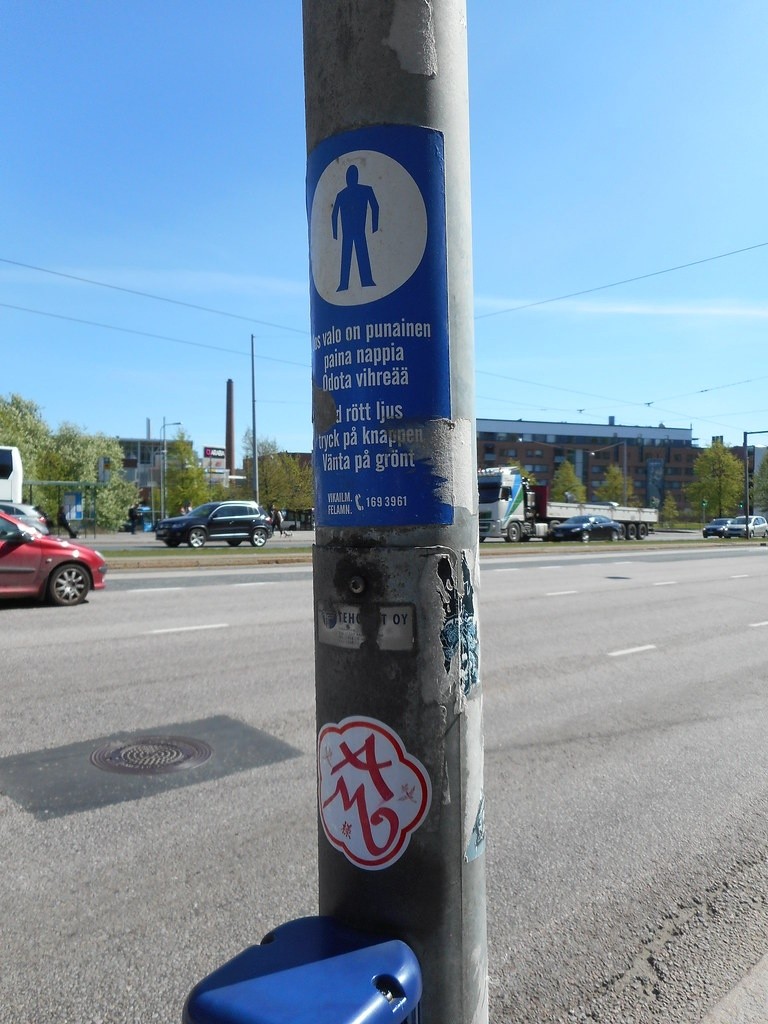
[550,515,627,542]
[703,517,734,538]
[0,510,108,607]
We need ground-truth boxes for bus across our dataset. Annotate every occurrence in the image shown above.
[0,445,24,506]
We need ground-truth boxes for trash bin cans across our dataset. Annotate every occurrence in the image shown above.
[179,915,426,1024]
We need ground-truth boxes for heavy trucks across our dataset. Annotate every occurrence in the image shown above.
[476,466,660,542]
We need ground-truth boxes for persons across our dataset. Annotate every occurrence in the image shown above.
[129,503,142,534]
[272,508,284,535]
[57,506,78,538]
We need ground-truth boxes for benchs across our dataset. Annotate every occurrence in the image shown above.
[49,525,69,535]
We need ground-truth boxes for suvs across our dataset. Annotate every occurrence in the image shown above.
[155,500,273,549]
[0,501,51,535]
[726,516,768,539]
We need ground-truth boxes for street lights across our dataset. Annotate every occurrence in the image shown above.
[161,422,182,520]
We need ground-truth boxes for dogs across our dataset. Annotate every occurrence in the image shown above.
[284,530,294,538]
